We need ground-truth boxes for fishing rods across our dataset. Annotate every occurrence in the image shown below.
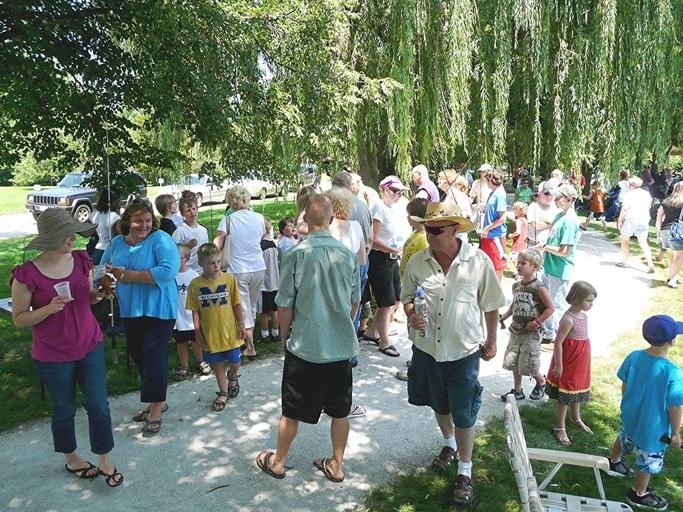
[439,159,506,329]
[106,119,119,365]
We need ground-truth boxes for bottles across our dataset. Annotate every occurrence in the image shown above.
[389,239,397,259]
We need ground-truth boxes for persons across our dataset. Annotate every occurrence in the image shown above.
[605,315,683,512]
[9,204,125,490]
[92,157,683,415]
[399,203,506,505]
[100,196,181,435]
[253,195,362,482]
[541,280,594,448]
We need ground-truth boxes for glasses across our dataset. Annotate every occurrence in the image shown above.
[391,189,404,194]
[131,199,153,208]
[424,224,457,236]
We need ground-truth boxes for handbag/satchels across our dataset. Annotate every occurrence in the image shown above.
[86,232,100,254]
[220,215,232,271]
[669,208,683,242]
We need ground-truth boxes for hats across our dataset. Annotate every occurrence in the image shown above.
[24,207,100,250]
[378,175,404,190]
[549,183,578,200]
[538,180,553,195]
[409,201,476,233]
[642,315,683,342]
[476,163,492,172]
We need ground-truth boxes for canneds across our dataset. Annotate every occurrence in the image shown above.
[92,265,106,295]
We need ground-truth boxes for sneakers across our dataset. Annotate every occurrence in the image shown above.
[256,335,271,344]
[529,375,547,400]
[625,487,668,510]
[396,371,408,381]
[606,456,634,476]
[347,402,367,418]
[501,388,525,401]
[269,333,280,341]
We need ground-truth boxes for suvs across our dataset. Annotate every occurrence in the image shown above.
[25,167,148,225]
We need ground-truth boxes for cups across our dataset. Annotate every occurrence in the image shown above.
[91,265,107,292]
[52,280,72,303]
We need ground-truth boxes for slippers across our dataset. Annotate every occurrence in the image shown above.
[362,331,380,344]
[313,457,344,482]
[99,466,124,487]
[256,450,286,478]
[133,404,169,422]
[141,416,162,433]
[66,461,99,479]
[379,345,400,356]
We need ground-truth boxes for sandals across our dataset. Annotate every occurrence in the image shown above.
[567,416,593,435]
[553,427,571,447]
[197,358,211,374]
[174,366,190,381]
[453,474,474,503]
[212,392,230,411]
[227,370,241,397]
[432,446,457,471]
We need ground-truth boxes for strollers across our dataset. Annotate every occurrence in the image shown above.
[605,186,620,223]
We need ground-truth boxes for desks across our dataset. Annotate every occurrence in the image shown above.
[0,296,134,399]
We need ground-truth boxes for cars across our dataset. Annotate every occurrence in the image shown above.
[281,163,320,191]
[223,168,287,199]
[164,171,228,208]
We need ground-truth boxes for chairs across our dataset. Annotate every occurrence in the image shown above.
[525,475,550,511]
[503,393,629,512]
